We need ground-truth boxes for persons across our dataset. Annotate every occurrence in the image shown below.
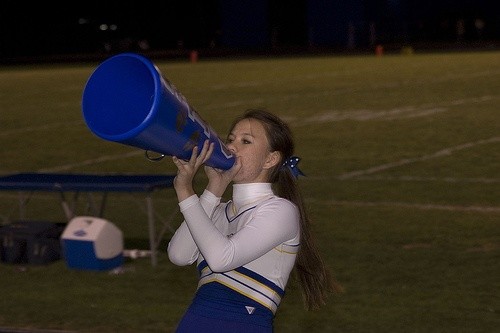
[165,107,344,333]
